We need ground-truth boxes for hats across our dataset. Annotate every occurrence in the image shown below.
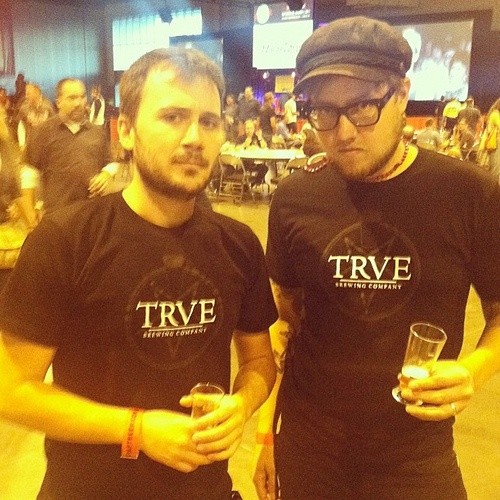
[293,16,413,86]
[465,95,474,101]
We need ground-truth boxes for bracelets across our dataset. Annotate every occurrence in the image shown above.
[121,407,144,461]
[257,432,278,446]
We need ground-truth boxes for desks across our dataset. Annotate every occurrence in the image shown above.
[239,150,294,159]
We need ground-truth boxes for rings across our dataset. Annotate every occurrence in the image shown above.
[450,402,457,415]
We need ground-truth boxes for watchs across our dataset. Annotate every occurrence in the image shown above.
[258,138,262,141]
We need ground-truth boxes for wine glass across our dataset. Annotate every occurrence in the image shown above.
[391,323,447,405]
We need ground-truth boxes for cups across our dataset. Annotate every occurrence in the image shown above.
[190,383,225,430]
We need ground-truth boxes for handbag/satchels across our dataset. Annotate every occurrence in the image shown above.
[478,126,499,150]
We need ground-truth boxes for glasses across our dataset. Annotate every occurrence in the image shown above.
[300,86,400,131]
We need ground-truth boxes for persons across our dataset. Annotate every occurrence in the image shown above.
[284,92,300,134]
[86,84,106,129]
[0,73,322,294]
[0,47,282,500]
[456,98,483,136]
[18,77,120,231]
[275,113,302,149]
[15,83,51,151]
[253,16,500,500]
[401,20,473,101]
[436,95,446,129]
[454,117,479,162]
[481,99,500,181]
[416,119,451,151]
[442,96,463,134]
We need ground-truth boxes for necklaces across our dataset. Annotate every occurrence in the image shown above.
[363,136,409,184]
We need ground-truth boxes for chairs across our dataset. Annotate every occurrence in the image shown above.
[218,154,255,205]
[270,159,306,202]
